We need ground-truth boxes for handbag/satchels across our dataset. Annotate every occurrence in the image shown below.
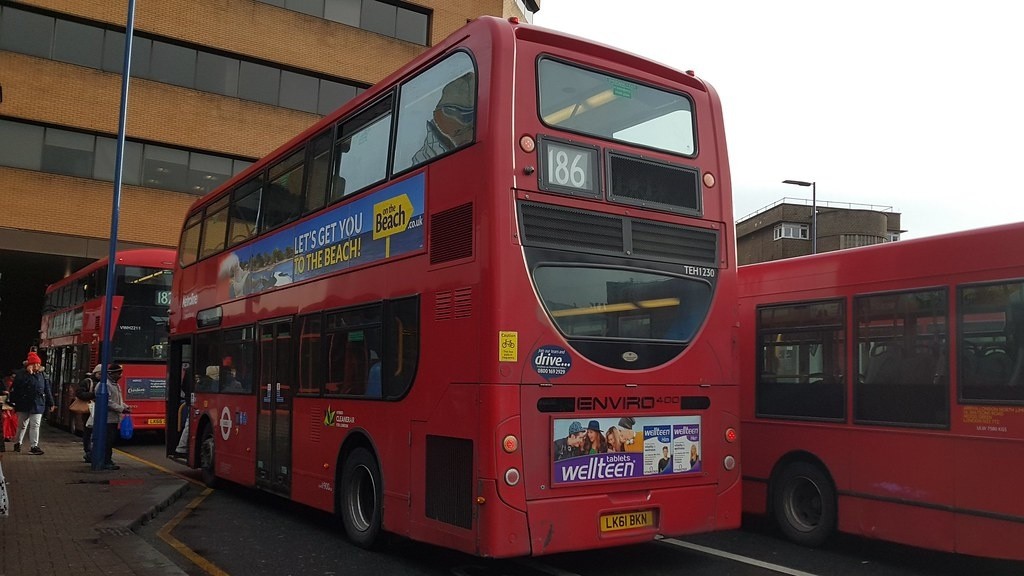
[3,409,18,437]
[120,415,133,439]
[69,378,93,413]
[86,400,96,425]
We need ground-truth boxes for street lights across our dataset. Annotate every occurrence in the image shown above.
[783,180,816,254]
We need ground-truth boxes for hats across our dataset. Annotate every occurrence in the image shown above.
[107,364,123,371]
[583,420,605,433]
[617,417,636,431]
[93,364,102,372]
[28,351,42,364]
[569,421,586,436]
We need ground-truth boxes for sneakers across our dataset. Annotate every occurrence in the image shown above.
[14,443,21,453]
[105,460,121,469]
[83,452,92,462]
[28,446,45,455]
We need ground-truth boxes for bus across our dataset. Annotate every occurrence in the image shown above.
[166,15,741,558]
[736,221,1024,559]
[550,297,680,338]
[38,249,197,435]
[775,312,1007,385]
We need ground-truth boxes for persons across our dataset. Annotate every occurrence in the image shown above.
[95,364,130,469]
[554,417,643,461]
[658,446,671,474]
[690,445,698,469]
[76,363,102,463]
[9,352,55,455]
[173,350,209,455]
[0,379,10,452]
